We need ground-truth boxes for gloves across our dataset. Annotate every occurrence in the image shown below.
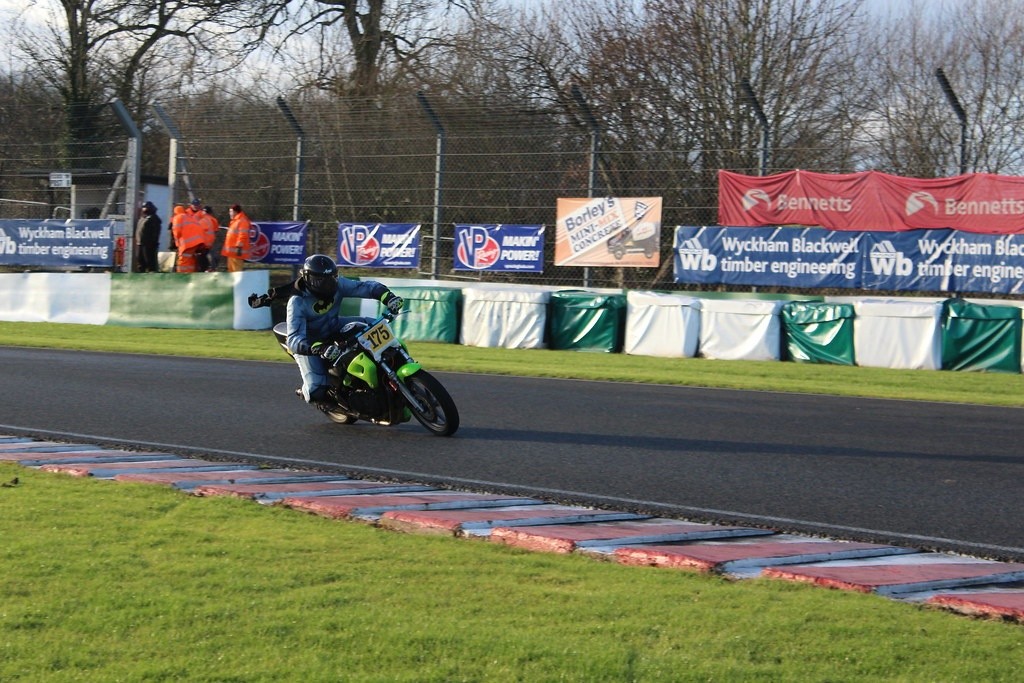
[380,290,404,313]
[311,341,339,362]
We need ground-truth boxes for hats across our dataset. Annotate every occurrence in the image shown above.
[230,203,241,212]
[141,201,152,208]
[174,206,184,214]
[202,206,212,213]
[191,199,200,206]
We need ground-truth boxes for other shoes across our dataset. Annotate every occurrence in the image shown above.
[199,266,216,272]
[138,265,159,273]
[310,388,338,412]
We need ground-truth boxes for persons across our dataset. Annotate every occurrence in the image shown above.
[134,200,150,272]
[169,197,219,273]
[140,204,161,272]
[219,203,251,272]
[285,253,404,411]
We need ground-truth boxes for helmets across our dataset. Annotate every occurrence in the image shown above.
[304,254,339,296]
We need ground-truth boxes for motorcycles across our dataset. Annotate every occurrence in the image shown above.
[274,303,460,437]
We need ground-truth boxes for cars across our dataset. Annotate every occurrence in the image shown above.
[608,226,659,261]
